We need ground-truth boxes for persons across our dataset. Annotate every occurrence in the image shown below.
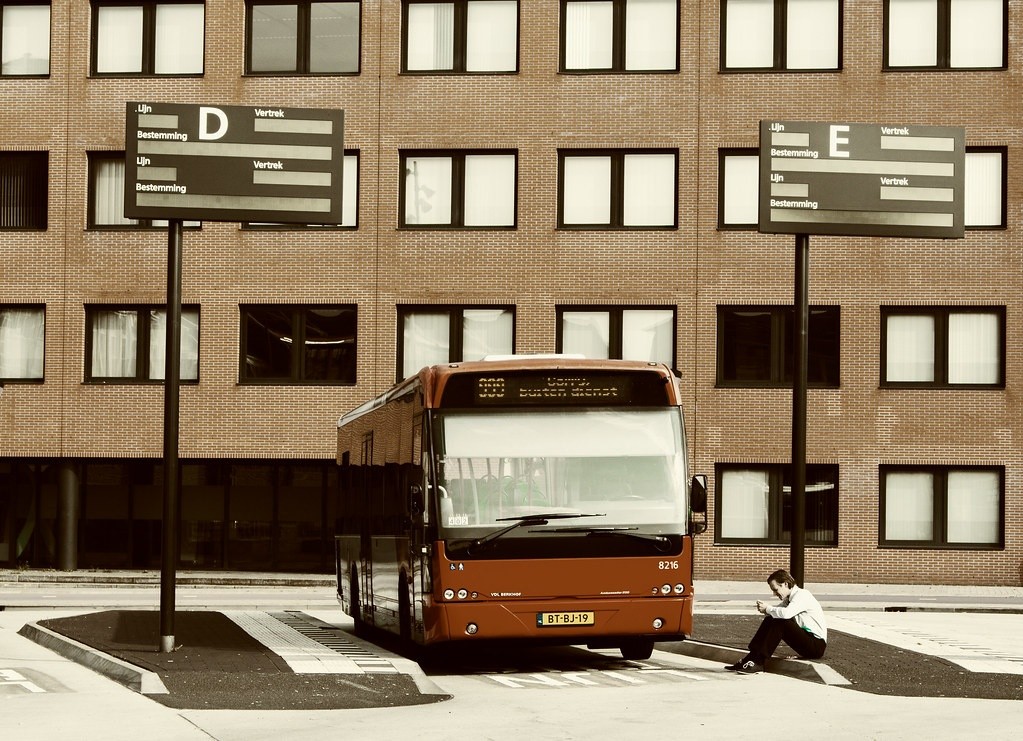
[724,569,827,675]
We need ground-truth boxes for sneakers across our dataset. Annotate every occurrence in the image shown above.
[737,660,763,674]
[723,659,747,671]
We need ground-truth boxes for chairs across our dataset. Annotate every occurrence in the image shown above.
[476,474,548,513]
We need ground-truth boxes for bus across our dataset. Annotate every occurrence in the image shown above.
[335,358,708,660]
